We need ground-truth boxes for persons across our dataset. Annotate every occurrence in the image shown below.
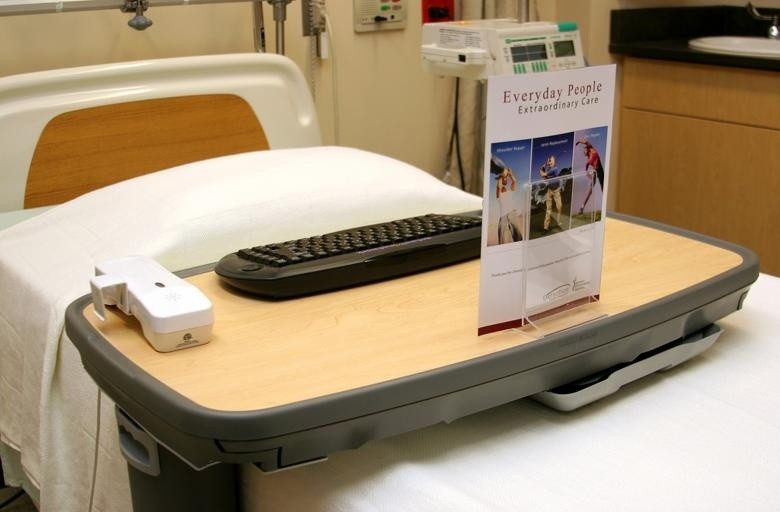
[576,141,604,215]
[538,156,564,235]
[495,168,525,245]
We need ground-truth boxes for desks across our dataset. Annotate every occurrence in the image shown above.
[56,197,766,490]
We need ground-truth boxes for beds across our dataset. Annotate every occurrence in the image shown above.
[0,44,779,512]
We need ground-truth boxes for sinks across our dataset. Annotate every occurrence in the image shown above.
[688,36,780,61]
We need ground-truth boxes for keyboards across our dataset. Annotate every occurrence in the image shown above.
[216,209,482,296]
